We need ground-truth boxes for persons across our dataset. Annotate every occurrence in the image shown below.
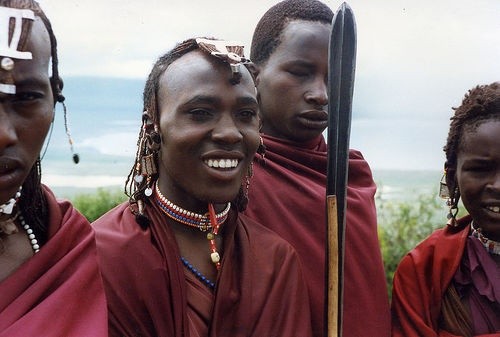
[391,81,500,337]
[90,36,312,337]
[0,0,108,337]
[244,0,392,337]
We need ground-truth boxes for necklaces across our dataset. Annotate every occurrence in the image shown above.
[471,220,500,254]
[153,179,234,274]
[0,185,42,254]
[181,257,216,290]
